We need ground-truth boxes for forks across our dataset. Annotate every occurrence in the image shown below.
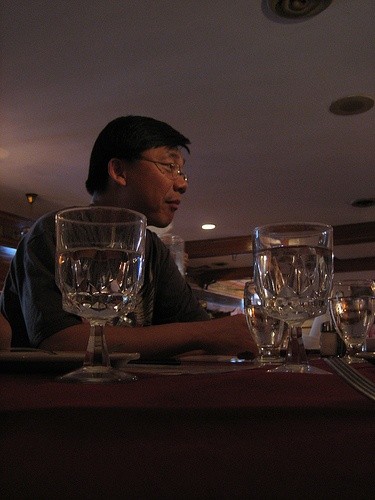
[327,356,375,402]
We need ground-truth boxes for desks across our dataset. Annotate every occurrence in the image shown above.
[0,355,375,500]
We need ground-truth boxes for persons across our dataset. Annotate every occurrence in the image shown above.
[0,115,284,358]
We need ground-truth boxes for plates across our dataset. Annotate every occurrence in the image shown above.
[354,351,375,365]
[0,349,142,365]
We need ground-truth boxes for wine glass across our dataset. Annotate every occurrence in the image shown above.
[330,278,375,365]
[55,205,148,386]
[244,280,286,366]
[250,223,335,375]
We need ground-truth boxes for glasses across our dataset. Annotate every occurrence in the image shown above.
[140,157,192,184]
